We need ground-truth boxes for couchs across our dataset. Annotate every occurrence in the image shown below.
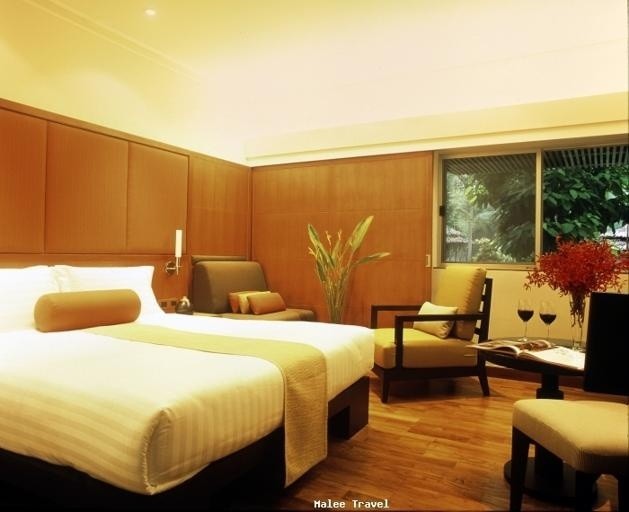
[194,260,315,321]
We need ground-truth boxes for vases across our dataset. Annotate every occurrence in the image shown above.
[568,291,586,351]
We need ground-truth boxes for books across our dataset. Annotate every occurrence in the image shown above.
[464,339,585,372]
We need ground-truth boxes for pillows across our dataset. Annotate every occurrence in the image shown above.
[229,290,287,315]
[412,300,459,340]
[0,264,165,333]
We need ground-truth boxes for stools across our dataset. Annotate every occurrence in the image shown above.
[507,400,629,512]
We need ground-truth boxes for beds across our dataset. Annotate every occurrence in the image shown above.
[0,311,375,512]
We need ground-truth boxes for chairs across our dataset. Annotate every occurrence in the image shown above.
[370,263,493,403]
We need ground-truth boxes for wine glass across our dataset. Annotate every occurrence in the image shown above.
[516,296,536,343]
[538,300,558,347]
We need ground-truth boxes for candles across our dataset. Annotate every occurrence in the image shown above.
[175,229,183,260]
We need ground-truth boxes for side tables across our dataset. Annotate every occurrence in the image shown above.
[478,338,604,507]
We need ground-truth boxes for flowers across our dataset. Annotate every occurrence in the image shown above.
[522,233,629,329]
